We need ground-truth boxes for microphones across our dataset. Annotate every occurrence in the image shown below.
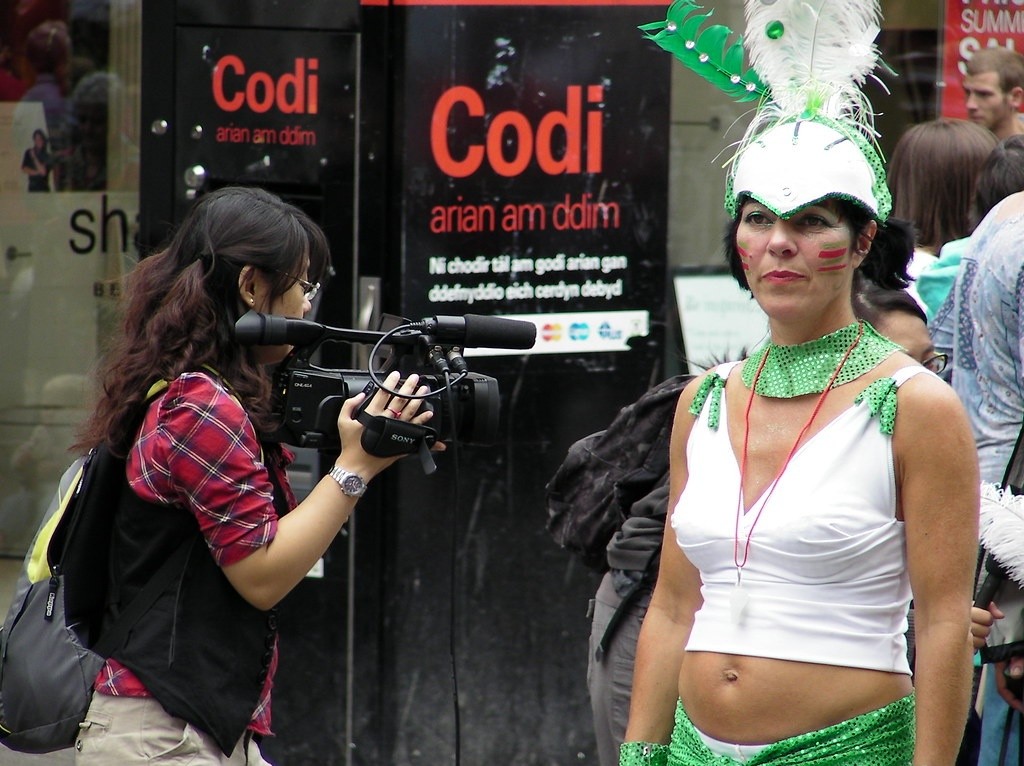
[425,315,537,349]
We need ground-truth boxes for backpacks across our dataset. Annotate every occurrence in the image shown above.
[0,361,245,754]
[542,373,700,573]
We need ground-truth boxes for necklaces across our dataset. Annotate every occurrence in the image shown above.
[723,320,862,624]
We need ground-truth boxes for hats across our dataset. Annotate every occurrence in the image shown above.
[637,1,894,225]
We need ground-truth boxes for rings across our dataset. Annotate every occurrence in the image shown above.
[388,407,401,418]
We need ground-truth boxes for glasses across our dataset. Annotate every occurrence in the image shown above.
[272,267,321,302]
[923,351,949,375]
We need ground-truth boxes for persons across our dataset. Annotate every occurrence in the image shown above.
[20,129,51,192]
[75,187,446,766]
[570,44,1024,766]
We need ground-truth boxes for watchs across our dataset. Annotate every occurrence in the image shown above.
[329,467,366,496]
